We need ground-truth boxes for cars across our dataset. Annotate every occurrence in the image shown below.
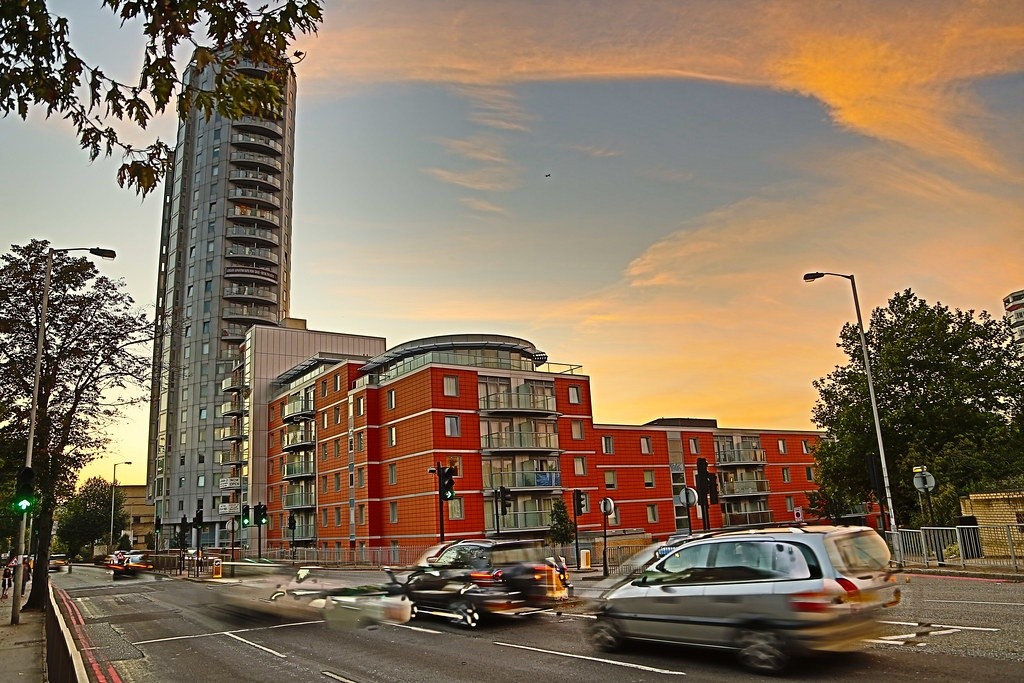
[48,554,62,573]
[593,525,901,674]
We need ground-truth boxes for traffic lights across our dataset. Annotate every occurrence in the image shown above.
[254,505,268,525]
[13,467,34,512]
[155,516,161,533]
[708,473,719,505]
[573,490,585,516]
[241,505,250,528]
[192,510,203,529]
[441,467,455,501]
[500,485,511,516]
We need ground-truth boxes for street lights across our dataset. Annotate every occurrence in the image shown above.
[804,272,906,563]
[11,248,116,626]
[110,461,132,552]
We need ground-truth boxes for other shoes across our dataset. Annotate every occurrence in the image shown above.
[22,594,25,597]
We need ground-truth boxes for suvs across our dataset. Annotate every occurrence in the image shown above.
[384,540,566,629]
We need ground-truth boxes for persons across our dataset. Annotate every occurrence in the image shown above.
[68,558,72,573]
[2,568,12,598]
[13,558,32,597]
